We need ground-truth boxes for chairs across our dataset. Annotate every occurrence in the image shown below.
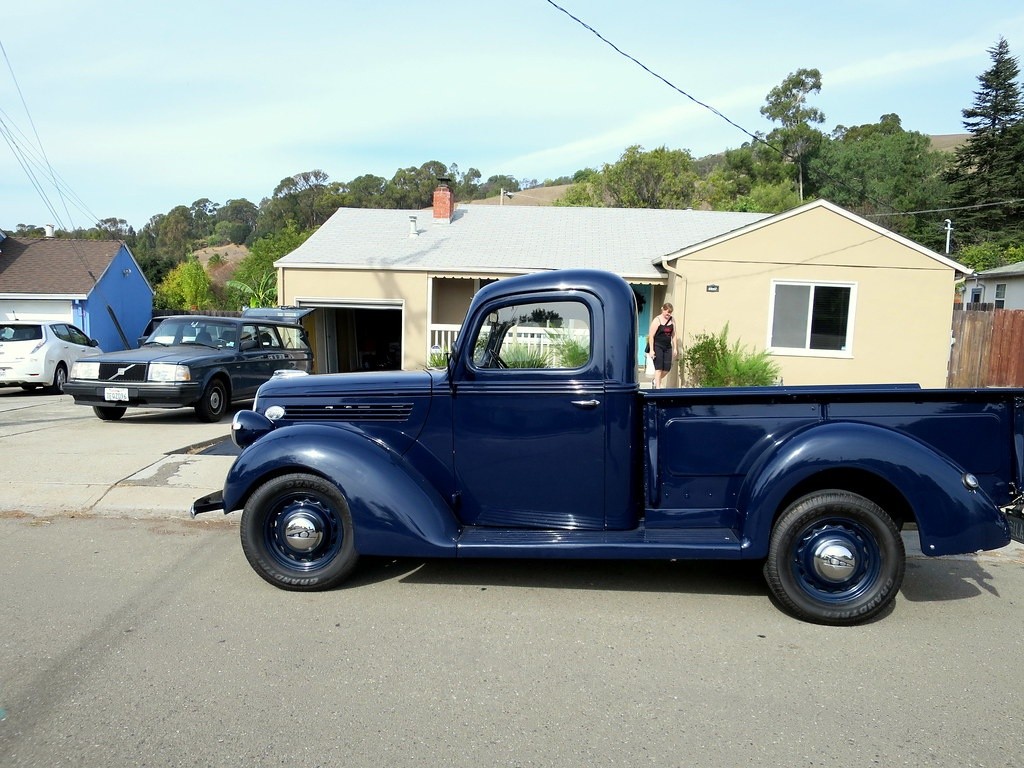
[221,331,238,343]
[13,331,25,338]
[196,332,212,343]
[241,332,251,342]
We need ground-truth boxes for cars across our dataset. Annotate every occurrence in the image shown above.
[136,315,235,349]
[0,321,107,395]
[60,306,317,424]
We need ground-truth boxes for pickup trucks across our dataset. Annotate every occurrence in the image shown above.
[191,267,1024,627]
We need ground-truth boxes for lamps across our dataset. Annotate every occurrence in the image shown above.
[706,284,720,293]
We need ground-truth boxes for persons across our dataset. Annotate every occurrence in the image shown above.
[649,303,677,388]
